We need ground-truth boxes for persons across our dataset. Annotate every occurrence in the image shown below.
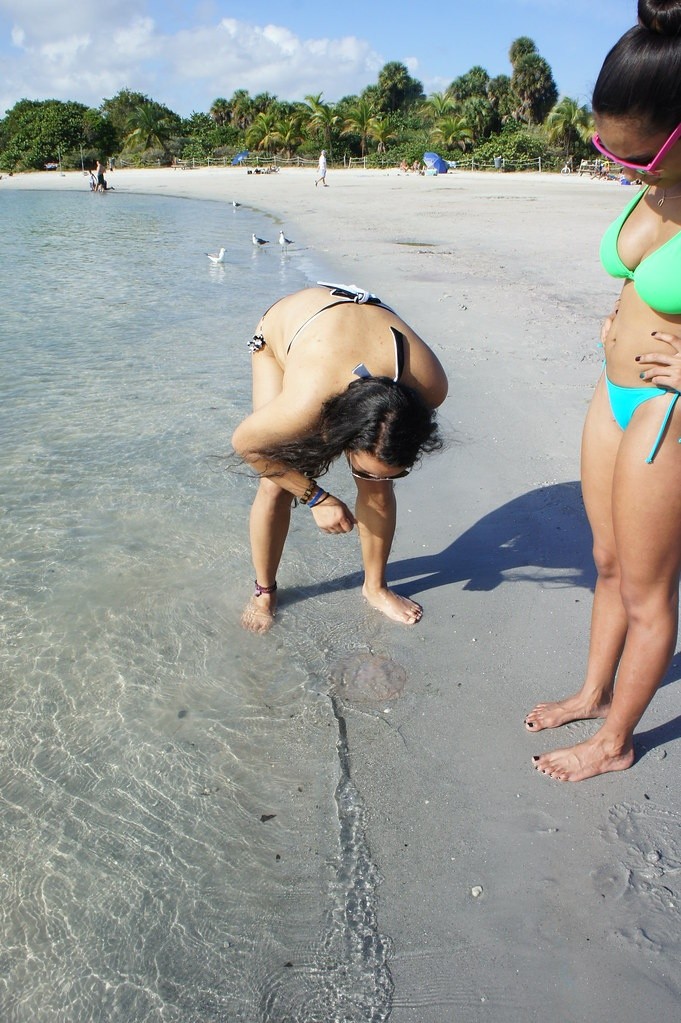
[413,159,425,176]
[520,0,681,786]
[87,159,116,194]
[590,155,642,186]
[314,149,330,188]
[204,280,450,638]
[399,158,409,172]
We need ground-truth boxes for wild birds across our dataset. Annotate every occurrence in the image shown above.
[203,247,228,263]
[232,200,242,207]
[252,233,270,247]
[279,230,295,248]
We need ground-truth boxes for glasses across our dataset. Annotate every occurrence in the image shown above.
[591,123,681,176]
[350,447,409,481]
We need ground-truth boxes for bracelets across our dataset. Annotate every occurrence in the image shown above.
[298,479,331,510]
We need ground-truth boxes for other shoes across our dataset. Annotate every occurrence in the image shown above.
[324,184,329,187]
[315,180,317,187]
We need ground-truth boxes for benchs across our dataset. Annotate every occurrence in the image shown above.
[577,159,610,177]
[170,160,189,170]
[45,162,57,171]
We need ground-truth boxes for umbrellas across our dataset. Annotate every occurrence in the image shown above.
[423,152,448,174]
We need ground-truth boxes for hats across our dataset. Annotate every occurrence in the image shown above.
[321,150,327,154]
